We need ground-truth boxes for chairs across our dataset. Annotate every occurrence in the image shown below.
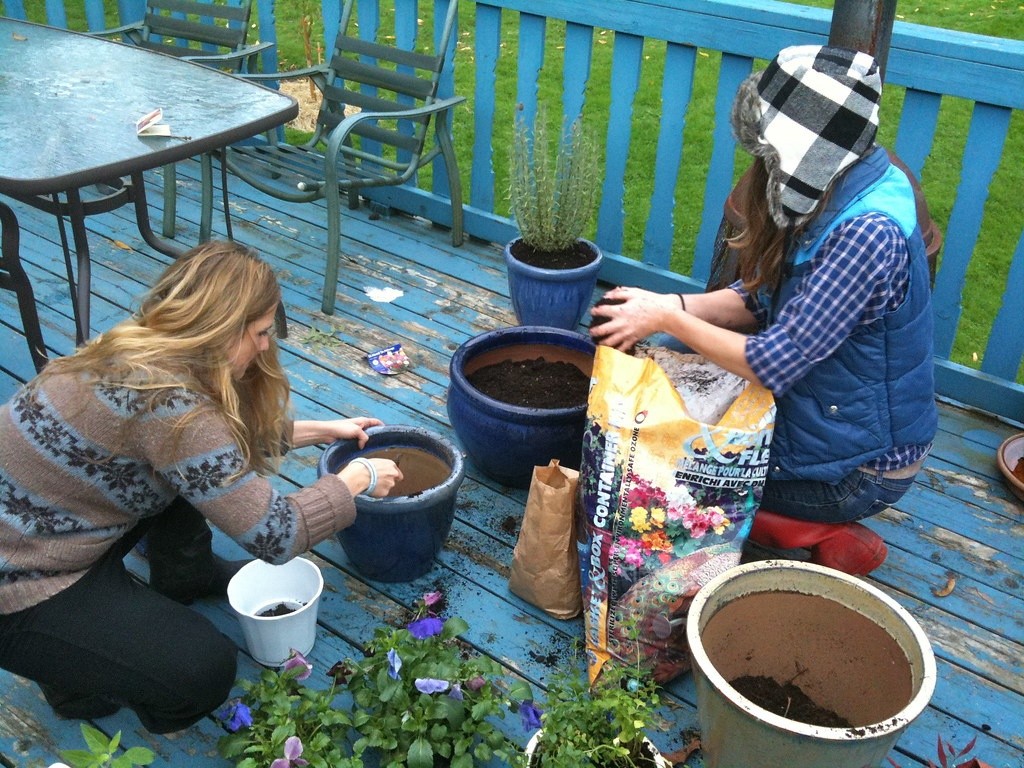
[199,0,464,313]
[81,0,281,239]
[0,203,46,376]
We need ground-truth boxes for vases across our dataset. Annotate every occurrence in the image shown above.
[227,557,325,669]
[686,559,939,768]
[447,326,603,488]
[315,424,462,585]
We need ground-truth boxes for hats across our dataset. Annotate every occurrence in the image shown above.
[730,43,883,230]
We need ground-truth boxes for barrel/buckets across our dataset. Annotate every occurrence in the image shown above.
[315,423,465,584]
[686,560,936,768]
[226,554,324,669]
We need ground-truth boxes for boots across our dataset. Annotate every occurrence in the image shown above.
[751,509,888,578]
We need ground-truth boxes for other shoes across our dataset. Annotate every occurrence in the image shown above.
[30,679,121,719]
[151,553,256,602]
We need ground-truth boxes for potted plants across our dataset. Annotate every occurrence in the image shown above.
[505,608,679,768]
[501,105,604,328]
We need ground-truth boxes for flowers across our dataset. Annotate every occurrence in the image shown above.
[54,589,531,768]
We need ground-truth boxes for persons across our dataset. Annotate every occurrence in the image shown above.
[0,240,407,734]
[589,46,936,577]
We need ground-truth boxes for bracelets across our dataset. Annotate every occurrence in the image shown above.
[671,291,686,311]
[348,456,377,494]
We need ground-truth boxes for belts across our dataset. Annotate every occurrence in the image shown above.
[858,457,924,483]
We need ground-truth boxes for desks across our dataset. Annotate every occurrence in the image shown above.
[0,14,299,354]
